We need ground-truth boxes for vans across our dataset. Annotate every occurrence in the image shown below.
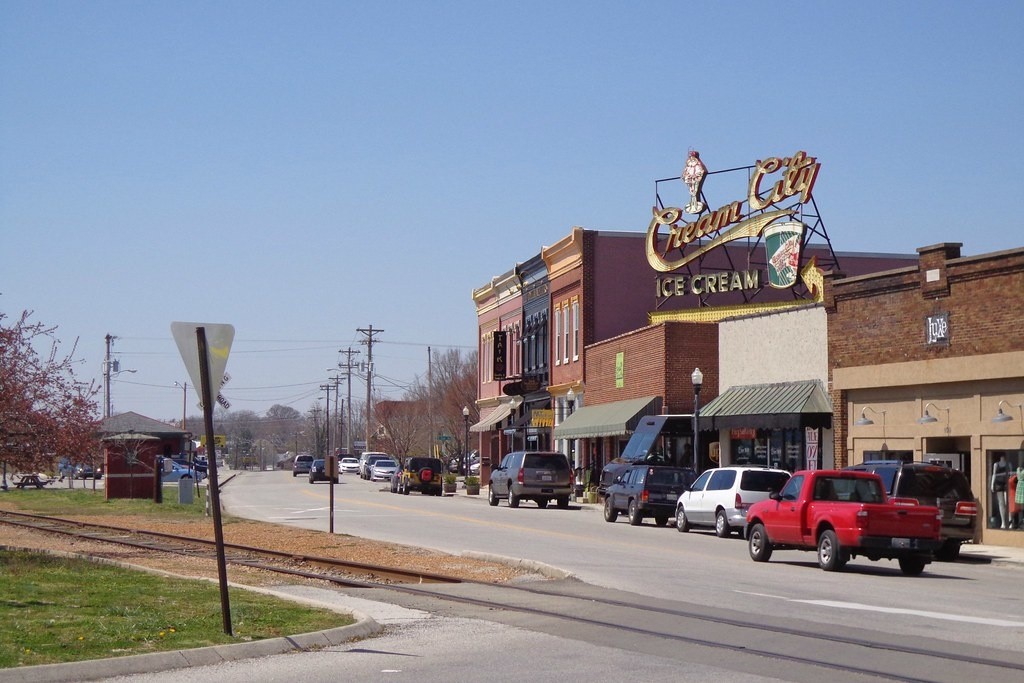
[596,415,718,496]
[675,466,799,539]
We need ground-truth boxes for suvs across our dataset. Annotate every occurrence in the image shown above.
[397,457,443,497]
[830,458,979,560]
[604,463,698,527]
[488,450,575,510]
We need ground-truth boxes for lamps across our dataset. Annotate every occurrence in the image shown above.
[856,406,886,426]
[921,402,951,422]
[992,401,1020,421]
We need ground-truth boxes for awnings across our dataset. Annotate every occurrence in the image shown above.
[469,398,550,433]
[691,384,833,432]
[553,396,658,442]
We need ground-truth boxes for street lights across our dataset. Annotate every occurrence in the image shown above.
[327,368,372,454]
[463,406,469,477]
[690,368,703,476]
[509,397,516,453]
[104,368,138,419]
[174,381,186,431]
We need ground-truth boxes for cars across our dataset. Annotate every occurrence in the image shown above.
[449,448,480,476]
[59,456,102,481]
[389,464,403,492]
[292,455,314,477]
[160,455,211,482]
[336,450,398,482]
[306,460,340,483]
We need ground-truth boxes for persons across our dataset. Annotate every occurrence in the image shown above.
[990,456,1024,531]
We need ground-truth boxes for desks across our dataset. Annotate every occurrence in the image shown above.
[17,474,43,489]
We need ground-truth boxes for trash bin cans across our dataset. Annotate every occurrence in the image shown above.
[178,479,194,504]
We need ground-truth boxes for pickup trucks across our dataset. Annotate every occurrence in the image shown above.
[744,470,943,575]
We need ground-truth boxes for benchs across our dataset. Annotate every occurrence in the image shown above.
[12,482,47,489]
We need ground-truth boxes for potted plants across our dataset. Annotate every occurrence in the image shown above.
[466,477,480,495]
[442,473,457,494]
